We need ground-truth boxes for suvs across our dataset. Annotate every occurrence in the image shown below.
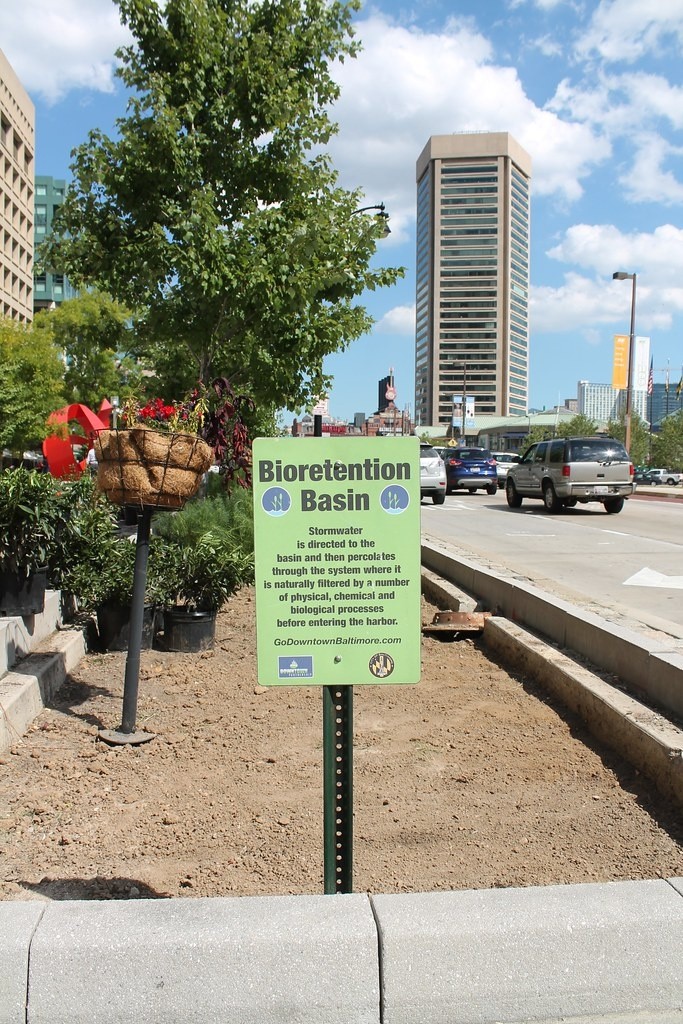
[420,441,520,504]
[506,435,637,514]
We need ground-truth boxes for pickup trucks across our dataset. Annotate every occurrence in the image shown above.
[646,469,683,485]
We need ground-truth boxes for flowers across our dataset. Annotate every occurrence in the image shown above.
[116,378,210,435]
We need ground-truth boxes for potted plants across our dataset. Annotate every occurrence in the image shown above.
[0,464,254,651]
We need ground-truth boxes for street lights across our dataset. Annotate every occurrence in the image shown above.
[613,271,636,453]
[453,361,466,439]
[526,416,530,442]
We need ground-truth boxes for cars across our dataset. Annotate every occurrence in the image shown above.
[633,472,662,486]
